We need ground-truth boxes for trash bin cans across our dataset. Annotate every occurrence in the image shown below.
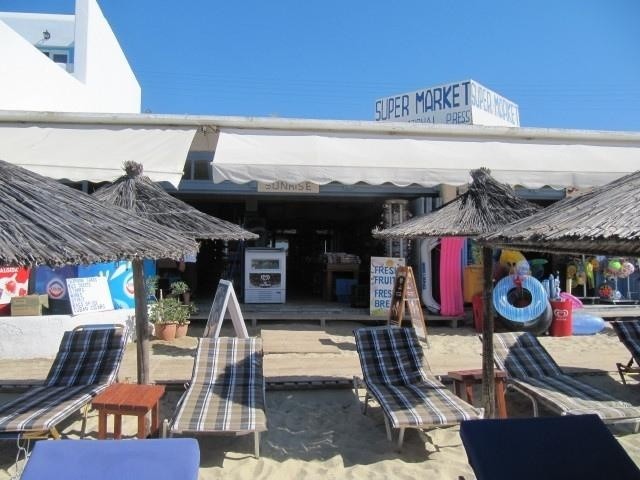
[472,293,483,333]
[550,300,573,336]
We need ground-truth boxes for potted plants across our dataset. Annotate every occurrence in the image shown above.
[145,274,197,340]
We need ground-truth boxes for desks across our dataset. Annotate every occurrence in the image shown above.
[447,368,508,421]
[321,264,360,302]
[92,383,166,442]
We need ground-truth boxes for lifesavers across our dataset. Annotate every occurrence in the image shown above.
[499,292,553,336]
[573,314,605,334]
[492,275,546,321]
[560,292,582,308]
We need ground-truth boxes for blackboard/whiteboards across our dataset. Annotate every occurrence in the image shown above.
[227,282,252,338]
[66,276,113,316]
[203,280,231,339]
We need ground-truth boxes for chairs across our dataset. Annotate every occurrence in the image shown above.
[609,321,640,373]
[0,316,129,460]
[17,438,203,479]
[479,332,640,438]
[352,325,480,454]
[457,413,640,480]
[168,336,265,459]
